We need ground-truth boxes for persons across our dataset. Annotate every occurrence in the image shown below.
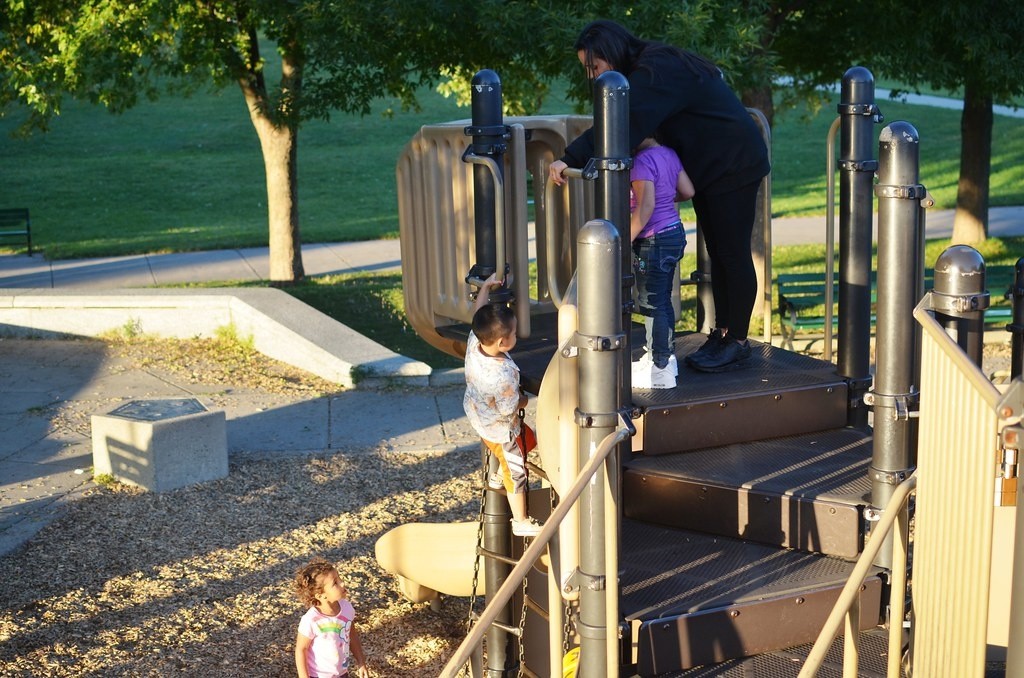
[463,271,546,536]
[295,556,369,678]
[631,136,698,391]
[548,17,774,371]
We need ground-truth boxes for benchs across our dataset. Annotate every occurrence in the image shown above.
[0,207,32,256]
[375,521,486,604]
[778,264,1019,356]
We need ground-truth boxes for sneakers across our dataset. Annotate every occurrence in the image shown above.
[488,471,503,490]
[639,346,678,377]
[509,516,543,536]
[631,361,676,389]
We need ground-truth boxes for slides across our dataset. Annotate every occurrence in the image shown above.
[373,519,486,598]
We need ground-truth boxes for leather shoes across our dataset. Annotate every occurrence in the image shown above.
[698,338,750,373]
[683,328,722,370]
